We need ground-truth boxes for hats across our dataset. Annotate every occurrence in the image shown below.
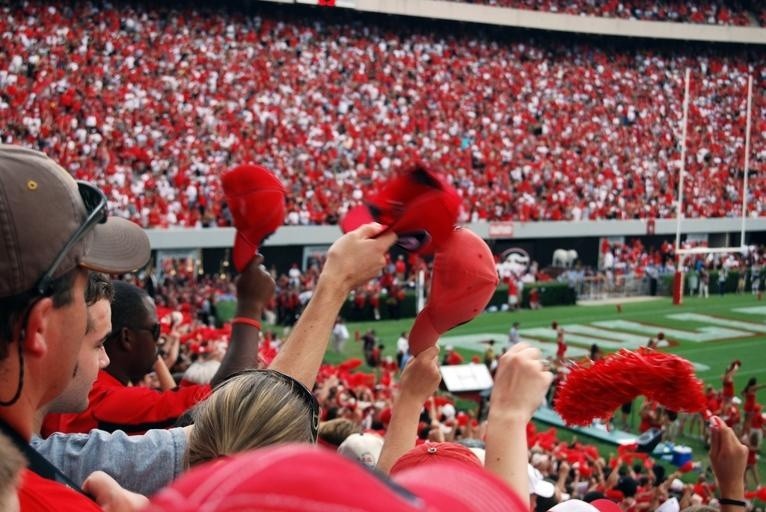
[390,463,531,512]
[390,441,482,477]
[144,442,419,512]
[220,164,287,272]
[0,144,151,300]
[408,224,500,357]
[339,163,461,256]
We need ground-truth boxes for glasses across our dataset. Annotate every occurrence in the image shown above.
[222,369,320,445]
[139,323,160,342]
[38,179,109,295]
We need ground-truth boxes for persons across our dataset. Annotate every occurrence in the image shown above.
[0,0,766,229]
[0,143,766,511]
